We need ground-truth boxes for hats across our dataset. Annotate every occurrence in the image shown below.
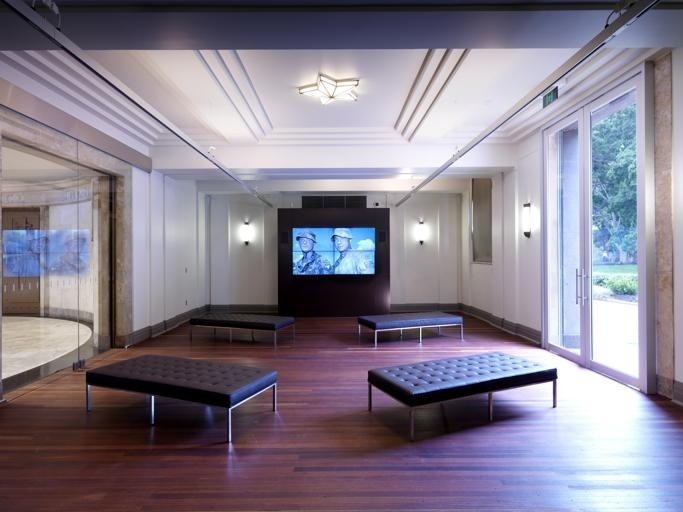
[64,230,87,245]
[26,232,49,242]
[296,231,317,244]
[330,228,352,242]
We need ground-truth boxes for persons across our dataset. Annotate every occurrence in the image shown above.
[49,232,88,274]
[11,233,49,274]
[329,228,372,274]
[292,231,332,274]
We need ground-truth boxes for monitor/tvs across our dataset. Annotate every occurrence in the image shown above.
[2,228,90,276]
[292,226,376,276]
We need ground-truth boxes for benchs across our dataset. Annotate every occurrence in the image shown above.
[354,308,466,351]
[184,309,298,351]
[363,351,562,443]
[81,349,280,447]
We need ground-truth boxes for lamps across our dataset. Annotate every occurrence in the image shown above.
[242,220,251,246]
[417,216,426,245]
[520,202,533,240]
[297,73,363,107]
